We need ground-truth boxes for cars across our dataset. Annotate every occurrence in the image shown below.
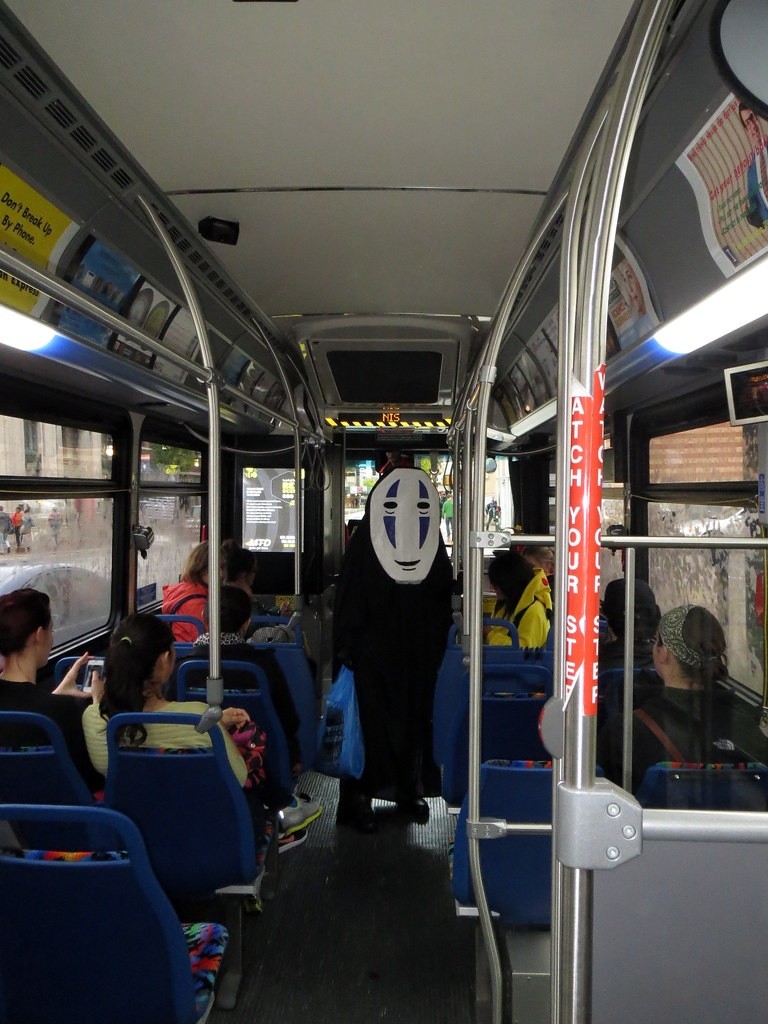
[672,506,761,538]
[0,562,110,651]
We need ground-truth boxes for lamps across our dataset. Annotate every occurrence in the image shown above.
[510,245,768,436]
[0,301,275,432]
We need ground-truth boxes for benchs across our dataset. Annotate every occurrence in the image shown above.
[432,620,768,1024]
[0,613,325,1024]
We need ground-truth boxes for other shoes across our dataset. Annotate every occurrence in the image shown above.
[349,789,378,835]
[400,797,429,815]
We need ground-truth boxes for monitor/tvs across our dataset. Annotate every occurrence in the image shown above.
[724,360,768,427]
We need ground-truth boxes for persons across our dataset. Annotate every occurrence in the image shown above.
[161,537,291,642]
[603,604,768,794]
[439,490,454,537]
[486,497,501,531]
[48,507,63,546]
[617,259,647,319]
[170,586,309,853]
[597,578,662,673]
[0,503,36,553]
[481,528,555,649]
[0,588,321,856]
[329,468,454,833]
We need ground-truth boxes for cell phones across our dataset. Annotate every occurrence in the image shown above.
[82,659,105,693]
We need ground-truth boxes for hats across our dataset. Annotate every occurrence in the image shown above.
[605,578,656,613]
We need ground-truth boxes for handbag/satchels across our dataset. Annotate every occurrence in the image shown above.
[312,666,365,780]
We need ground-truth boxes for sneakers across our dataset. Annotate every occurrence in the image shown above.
[279,792,323,837]
[277,827,309,854]
